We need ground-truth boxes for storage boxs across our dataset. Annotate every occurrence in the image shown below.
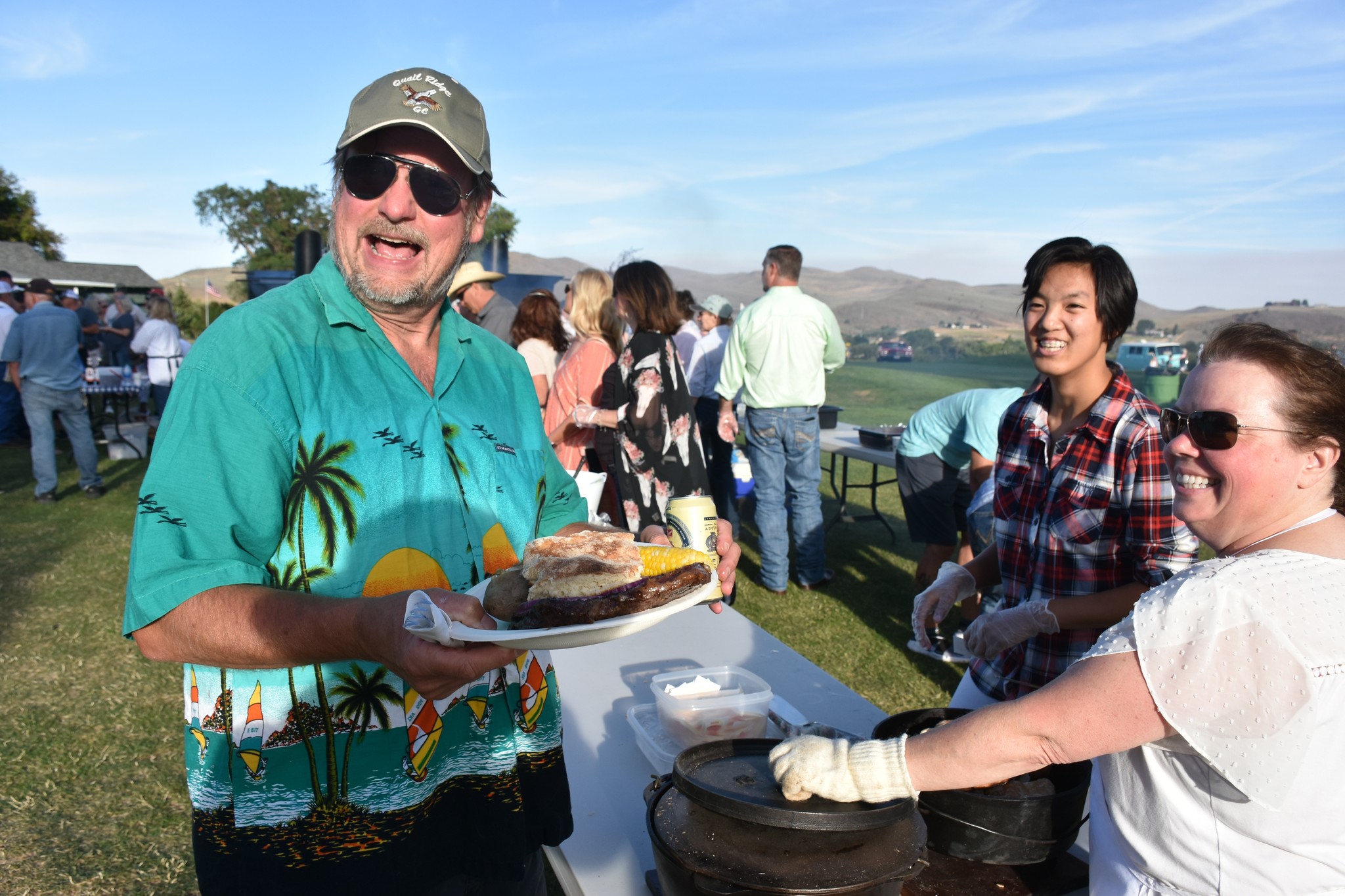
[102,421,150,461]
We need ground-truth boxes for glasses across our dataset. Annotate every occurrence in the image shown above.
[612,286,618,298]
[337,145,480,218]
[1158,407,1304,451]
[564,284,571,293]
[456,287,469,301]
[699,312,704,316]
[61,295,67,298]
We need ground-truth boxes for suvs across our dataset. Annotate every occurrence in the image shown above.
[875,341,913,362]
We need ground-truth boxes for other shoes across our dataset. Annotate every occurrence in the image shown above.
[33,489,57,504]
[86,485,105,498]
[800,570,832,590]
[105,405,115,413]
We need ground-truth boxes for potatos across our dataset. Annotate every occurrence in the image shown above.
[483,569,532,622]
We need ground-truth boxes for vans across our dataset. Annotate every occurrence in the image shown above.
[1117,342,1187,372]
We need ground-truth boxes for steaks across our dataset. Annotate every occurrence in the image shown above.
[508,562,712,630]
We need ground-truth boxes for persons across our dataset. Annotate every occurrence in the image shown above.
[714,243,846,594]
[767,322,1344,896]
[0,276,106,502]
[894,385,1031,667]
[446,261,520,347]
[507,260,737,547]
[117,64,738,896]
[1,269,193,448]
[130,297,184,454]
[938,235,1200,715]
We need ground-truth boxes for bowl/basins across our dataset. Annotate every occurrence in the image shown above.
[626,702,787,775]
[650,665,774,751]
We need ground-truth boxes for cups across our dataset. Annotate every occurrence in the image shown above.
[892,436,900,452]
[132,373,142,387]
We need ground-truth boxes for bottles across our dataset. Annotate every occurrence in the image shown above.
[122,363,131,387]
[92,357,100,384]
[84,358,95,385]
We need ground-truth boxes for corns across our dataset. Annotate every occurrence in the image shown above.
[638,546,714,577]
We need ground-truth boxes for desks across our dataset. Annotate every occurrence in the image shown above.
[545,601,889,895]
[83,385,148,461]
[819,422,900,545]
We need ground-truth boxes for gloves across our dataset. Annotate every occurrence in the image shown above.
[768,730,921,803]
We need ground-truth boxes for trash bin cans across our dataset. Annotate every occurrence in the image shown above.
[1142,367,1181,408]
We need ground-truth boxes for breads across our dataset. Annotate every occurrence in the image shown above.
[522,529,642,601]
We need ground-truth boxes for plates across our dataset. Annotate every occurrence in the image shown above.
[447,541,718,651]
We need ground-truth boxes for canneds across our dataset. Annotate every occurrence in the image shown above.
[665,495,723,606]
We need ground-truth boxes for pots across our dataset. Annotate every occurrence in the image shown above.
[871,707,1094,865]
[642,771,930,896]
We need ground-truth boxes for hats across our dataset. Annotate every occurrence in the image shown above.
[0,280,25,294]
[336,66,495,180]
[114,286,129,294]
[61,288,80,300]
[19,279,55,295]
[446,261,506,303]
[688,294,733,320]
[149,288,164,298]
